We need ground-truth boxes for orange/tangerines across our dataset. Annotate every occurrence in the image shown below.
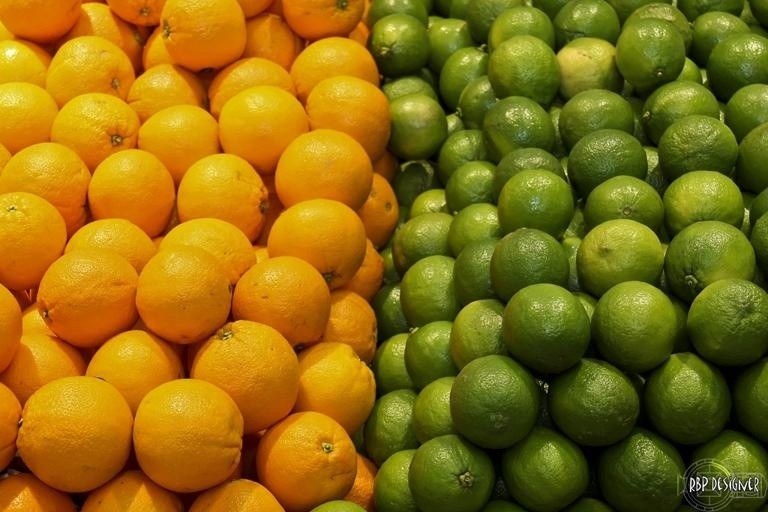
[0,0,767,512]
